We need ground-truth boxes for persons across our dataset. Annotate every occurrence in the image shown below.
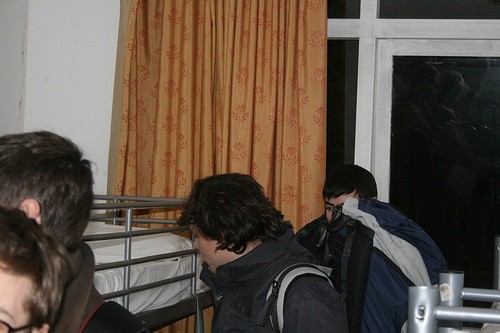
[0,205,68,333]
[174,172,349,333]
[295,164,451,333]
[0,130,149,332]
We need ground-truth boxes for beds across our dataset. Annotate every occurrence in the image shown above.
[81,221,213,319]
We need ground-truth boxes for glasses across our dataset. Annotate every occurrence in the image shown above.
[0,320,37,333]
